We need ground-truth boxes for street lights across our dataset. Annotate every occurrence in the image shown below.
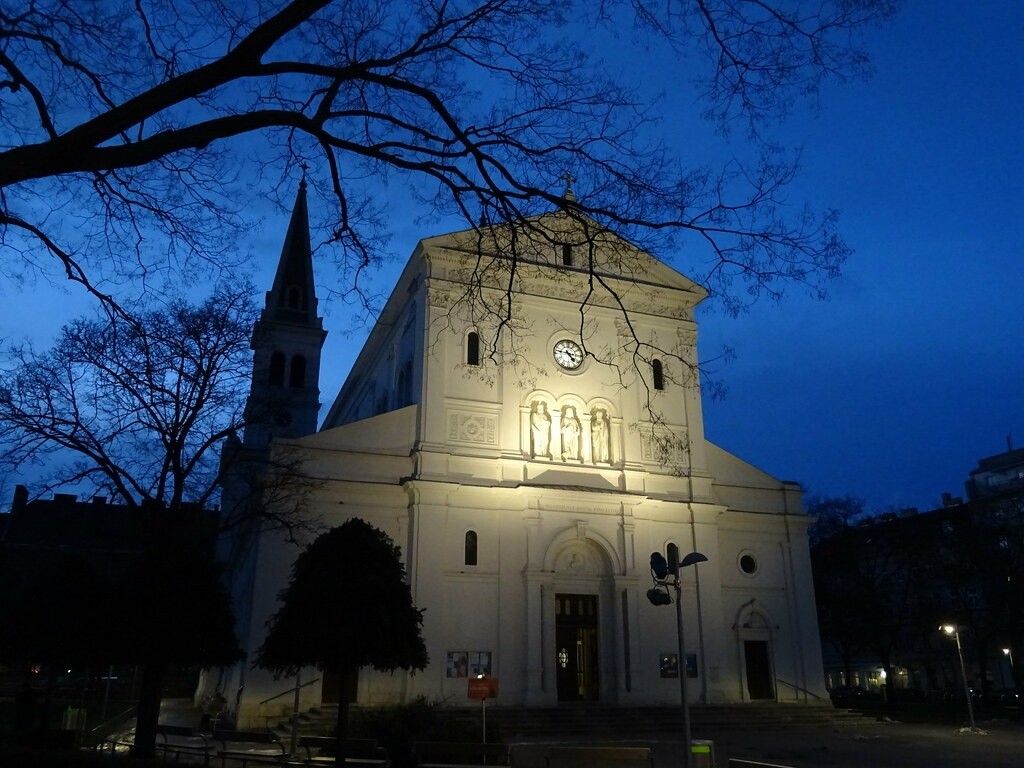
[1002,646,1015,668]
[647,541,709,768]
[939,622,977,733]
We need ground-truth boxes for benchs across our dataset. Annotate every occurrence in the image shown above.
[88,723,656,768]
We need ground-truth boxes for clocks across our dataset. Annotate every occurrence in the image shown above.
[553,340,583,370]
